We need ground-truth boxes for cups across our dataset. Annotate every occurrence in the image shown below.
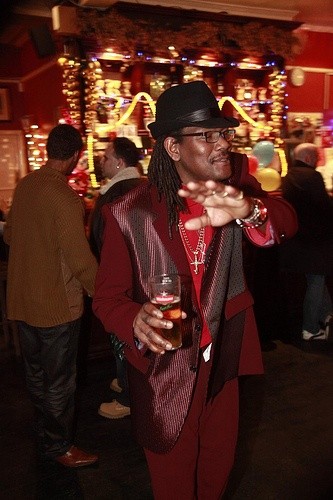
[149,273,182,349]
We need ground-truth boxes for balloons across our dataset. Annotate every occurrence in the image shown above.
[255,168,281,192]
[247,156,258,172]
[252,141,275,167]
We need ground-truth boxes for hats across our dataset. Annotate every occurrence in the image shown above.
[147,81,241,139]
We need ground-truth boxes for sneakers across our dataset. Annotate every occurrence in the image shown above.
[109,378,123,393]
[98,400,132,419]
[304,330,327,339]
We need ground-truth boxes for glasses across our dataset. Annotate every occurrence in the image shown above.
[175,129,236,143]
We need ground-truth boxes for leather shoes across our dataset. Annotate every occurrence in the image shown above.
[54,445,98,468]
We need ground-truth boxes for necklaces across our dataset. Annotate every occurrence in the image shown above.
[178,210,206,274]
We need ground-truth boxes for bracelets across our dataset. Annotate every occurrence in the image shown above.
[236,199,267,227]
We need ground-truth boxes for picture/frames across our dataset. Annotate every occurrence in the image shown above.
[0,85,14,123]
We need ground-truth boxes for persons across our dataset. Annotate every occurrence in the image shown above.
[92,82,299,500]
[284,143,332,345]
[0,124,144,468]
